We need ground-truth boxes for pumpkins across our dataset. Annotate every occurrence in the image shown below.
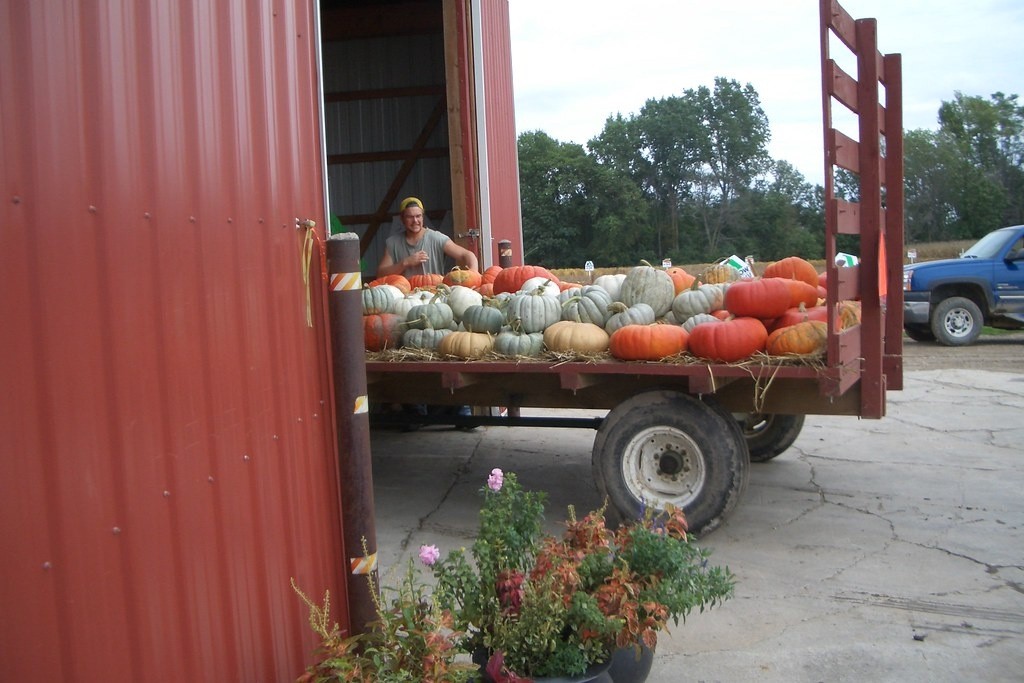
[360,256,861,360]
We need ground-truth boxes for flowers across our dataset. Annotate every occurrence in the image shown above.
[290,468,738,683]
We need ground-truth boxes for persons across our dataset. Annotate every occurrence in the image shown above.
[376,196,478,431]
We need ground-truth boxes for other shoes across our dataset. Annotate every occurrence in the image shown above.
[400,423,418,431]
[454,423,480,429]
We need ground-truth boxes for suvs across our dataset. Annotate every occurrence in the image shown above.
[901,225,1024,347]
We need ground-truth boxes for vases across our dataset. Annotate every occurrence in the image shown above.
[534,635,653,683]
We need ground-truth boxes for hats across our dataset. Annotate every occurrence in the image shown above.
[399,197,423,215]
